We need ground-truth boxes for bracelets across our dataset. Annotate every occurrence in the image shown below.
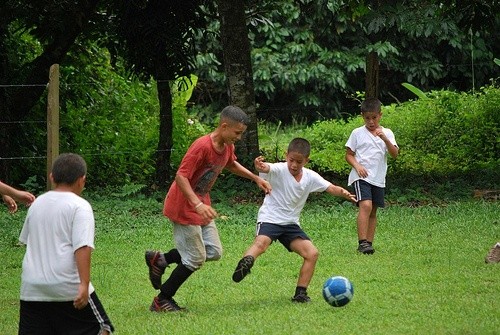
[195,203,203,209]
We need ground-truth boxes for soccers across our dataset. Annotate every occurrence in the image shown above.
[322,275,355,307]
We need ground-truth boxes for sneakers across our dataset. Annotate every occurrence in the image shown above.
[358,242,375,255]
[232,255,254,282]
[149,297,188,312]
[145,249,169,291]
[291,293,311,303]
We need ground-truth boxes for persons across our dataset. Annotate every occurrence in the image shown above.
[345,98,399,255]
[18,154,115,335]
[232,138,357,303]
[145,105,273,313]
[0,181,35,214]
